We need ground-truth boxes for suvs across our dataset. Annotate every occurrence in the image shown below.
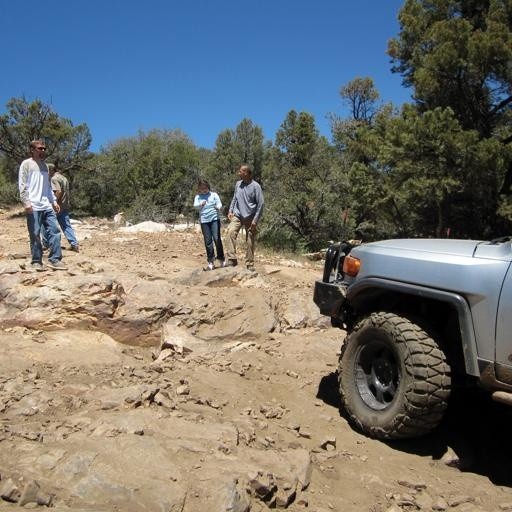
[310,208,512,443]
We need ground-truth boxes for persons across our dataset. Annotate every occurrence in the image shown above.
[19,141,68,271]
[47,163,80,253]
[193,180,225,270]
[227,166,264,266]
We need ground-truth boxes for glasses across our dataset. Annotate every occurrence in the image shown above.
[37,147,46,151]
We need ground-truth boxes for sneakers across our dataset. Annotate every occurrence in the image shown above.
[207,260,237,270]
[32,262,43,271]
[248,265,255,271]
[47,260,69,270]
